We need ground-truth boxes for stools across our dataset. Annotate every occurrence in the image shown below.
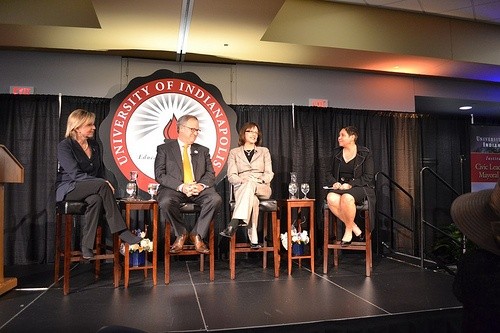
[324,199,374,276]
[54,162,121,296]
[163,203,216,284]
[229,183,281,279]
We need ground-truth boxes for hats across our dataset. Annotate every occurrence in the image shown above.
[450,180,500,256]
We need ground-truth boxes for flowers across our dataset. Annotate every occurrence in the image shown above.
[120,228,154,255]
[280,226,310,250]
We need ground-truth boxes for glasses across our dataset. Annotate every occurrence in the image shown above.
[244,130,259,135]
[180,124,201,134]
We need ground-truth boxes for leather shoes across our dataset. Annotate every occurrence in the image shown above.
[340,225,365,247]
[188,232,210,255]
[168,229,189,254]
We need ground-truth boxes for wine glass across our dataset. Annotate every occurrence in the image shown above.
[126,183,135,200]
[147,183,158,201]
[301,183,310,199]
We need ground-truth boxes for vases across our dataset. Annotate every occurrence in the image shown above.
[129,251,147,266]
[292,242,304,256]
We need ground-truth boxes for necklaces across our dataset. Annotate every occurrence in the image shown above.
[244,146,254,156]
[83,140,88,151]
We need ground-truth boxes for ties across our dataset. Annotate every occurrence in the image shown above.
[183,145,194,184]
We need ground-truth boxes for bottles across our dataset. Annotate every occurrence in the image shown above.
[130,171,139,199]
[289,172,299,200]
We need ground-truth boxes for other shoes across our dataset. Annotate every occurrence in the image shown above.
[219,228,233,238]
[118,230,142,244]
[81,246,94,259]
[250,242,262,249]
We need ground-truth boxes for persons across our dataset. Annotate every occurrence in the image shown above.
[219,121,274,249]
[55,108,143,259]
[450,181,500,333]
[323,126,377,246]
[153,115,222,254]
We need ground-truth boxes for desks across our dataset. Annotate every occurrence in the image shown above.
[118,200,158,288]
[279,198,316,276]
